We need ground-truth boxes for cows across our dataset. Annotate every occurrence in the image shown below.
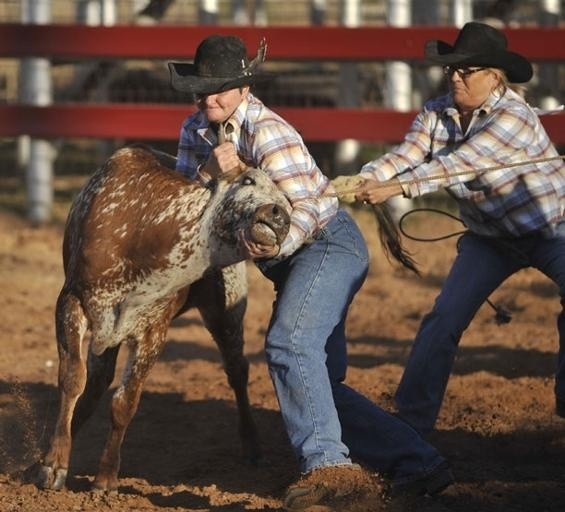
[36,143,423,501]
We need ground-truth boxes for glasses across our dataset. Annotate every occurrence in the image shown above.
[439,62,491,78]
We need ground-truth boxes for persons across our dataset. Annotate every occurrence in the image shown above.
[351,19,565,440]
[168,31,460,507]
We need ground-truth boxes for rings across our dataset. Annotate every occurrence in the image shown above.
[362,201,367,206]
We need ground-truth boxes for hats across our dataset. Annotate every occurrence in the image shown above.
[424,21,534,84]
[166,33,268,96]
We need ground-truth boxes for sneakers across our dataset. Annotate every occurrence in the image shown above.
[283,483,332,509]
[390,461,456,503]
